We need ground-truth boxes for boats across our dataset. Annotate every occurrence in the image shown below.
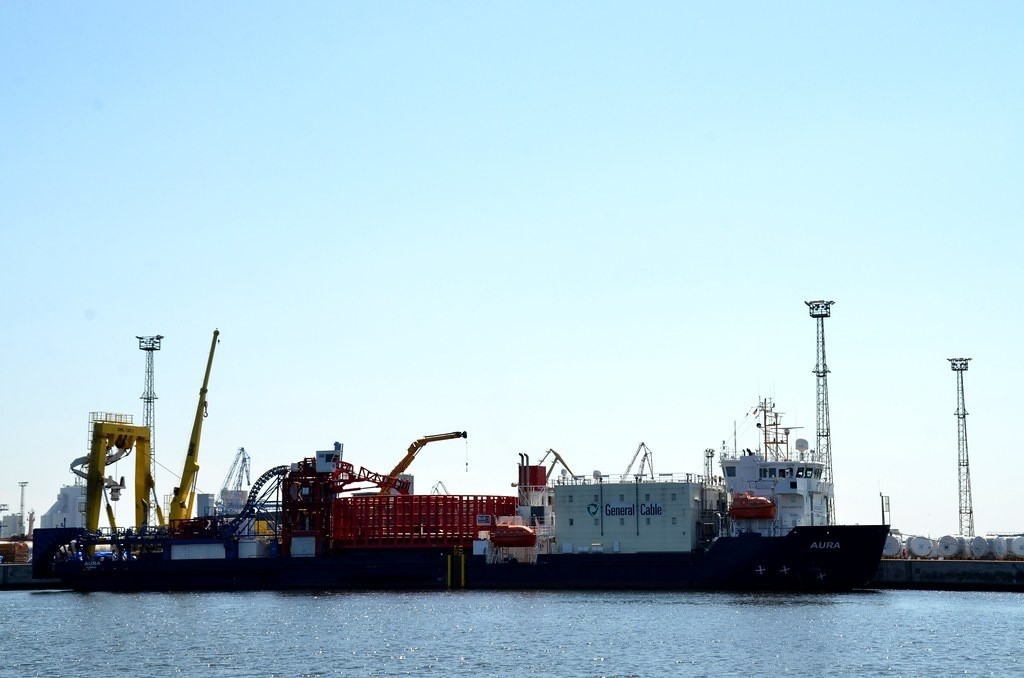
[482,395,890,592]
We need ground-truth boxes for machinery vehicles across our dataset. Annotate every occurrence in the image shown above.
[350,431,467,537]
